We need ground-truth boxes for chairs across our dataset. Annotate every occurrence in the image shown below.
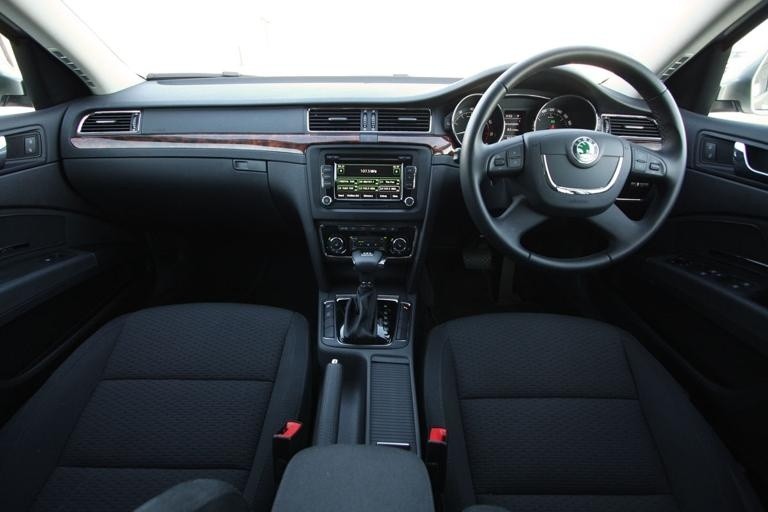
[0,299,317,512]
[416,306,762,511]
